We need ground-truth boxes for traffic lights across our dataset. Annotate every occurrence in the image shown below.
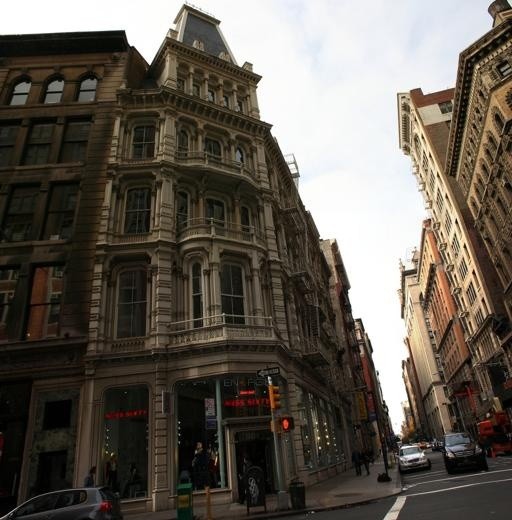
[270,386,283,408]
[282,416,294,432]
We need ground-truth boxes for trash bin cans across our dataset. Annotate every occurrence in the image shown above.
[288,482,305,509]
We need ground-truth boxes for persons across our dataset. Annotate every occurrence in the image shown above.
[206,439,219,488]
[350,445,362,477]
[106,452,118,493]
[361,447,370,475]
[244,454,268,507]
[83,465,97,488]
[236,459,245,504]
[191,440,210,491]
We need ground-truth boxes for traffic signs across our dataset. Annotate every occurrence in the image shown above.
[256,369,279,377]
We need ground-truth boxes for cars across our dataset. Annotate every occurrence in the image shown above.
[1,487,122,520]
[395,432,488,474]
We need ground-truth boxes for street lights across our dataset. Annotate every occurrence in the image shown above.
[367,391,388,469]
